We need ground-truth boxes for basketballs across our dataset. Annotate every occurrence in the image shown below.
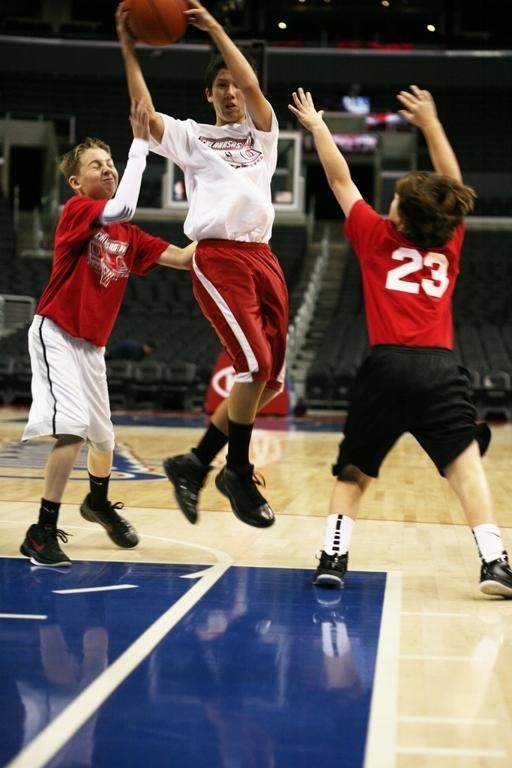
[125,2,188,47]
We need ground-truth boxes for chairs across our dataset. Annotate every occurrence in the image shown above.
[2,295,38,405]
[450,295,512,427]
[305,295,367,407]
[104,296,221,413]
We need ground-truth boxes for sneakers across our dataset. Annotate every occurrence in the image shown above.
[79,562,133,586]
[479,550,512,597]
[214,464,275,528]
[80,492,139,548]
[20,524,73,567]
[30,566,72,584]
[311,550,348,586]
[312,587,349,625]
[163,452,209,525]
